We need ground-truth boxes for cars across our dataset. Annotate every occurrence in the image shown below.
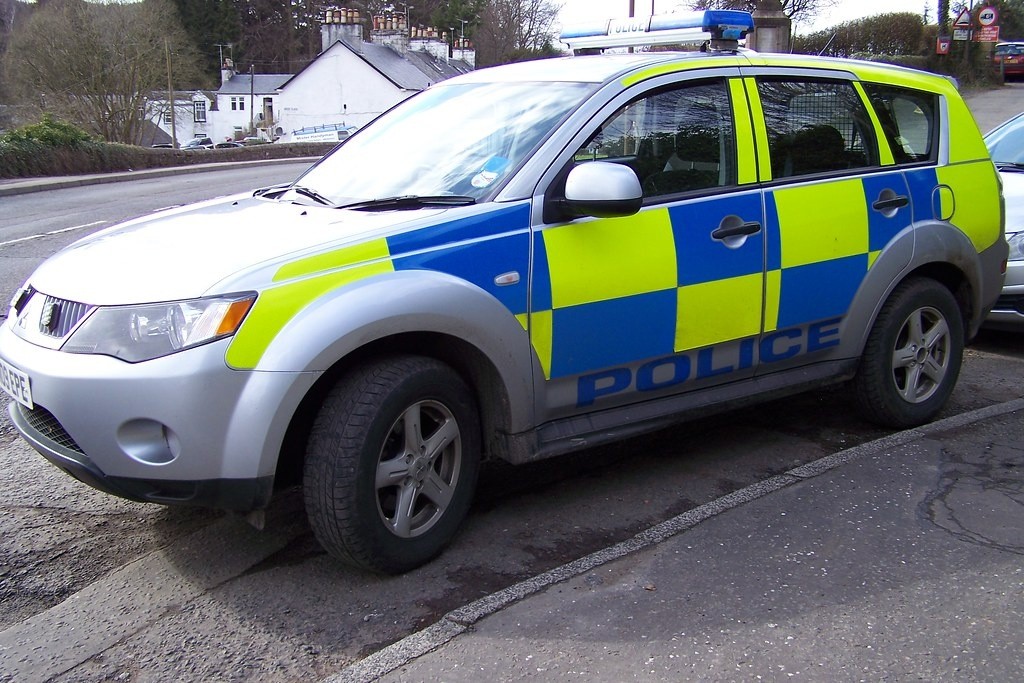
[972,112,1024,333]
[151,136,274,150]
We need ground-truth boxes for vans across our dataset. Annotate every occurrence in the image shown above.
[271,122,359,147]
[992,42,1024,80]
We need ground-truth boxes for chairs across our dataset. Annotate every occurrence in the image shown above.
[643,103,732,198]
[770,126,856,177]
[628,116,680,177]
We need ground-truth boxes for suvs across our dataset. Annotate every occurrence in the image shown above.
[0,11,1012,572]
